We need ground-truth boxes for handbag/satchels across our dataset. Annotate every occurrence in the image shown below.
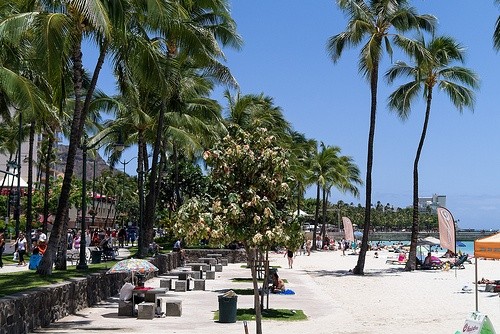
[13,252,19,261]
[485,283,500,293]
[69,234,73,243]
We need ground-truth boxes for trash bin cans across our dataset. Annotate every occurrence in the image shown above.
[266,269,277,282]
[218,294,239,322]
[91,250,103,265]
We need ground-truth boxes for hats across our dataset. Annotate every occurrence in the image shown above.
[67,229,72,232]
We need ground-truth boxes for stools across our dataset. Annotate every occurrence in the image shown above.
[118,259,228,319]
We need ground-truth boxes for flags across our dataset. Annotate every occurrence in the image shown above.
[342,217,355,244]
[437,207,457,253]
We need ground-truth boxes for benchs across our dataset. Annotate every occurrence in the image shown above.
[67,244,118,266]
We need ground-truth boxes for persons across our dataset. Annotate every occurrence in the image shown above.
[119,279,146,303]
[0,225,500,293]
[133,280,145,297]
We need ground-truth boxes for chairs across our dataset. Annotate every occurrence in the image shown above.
[416,257,430,270]
[265,269,276,288]
[450,254,468,269]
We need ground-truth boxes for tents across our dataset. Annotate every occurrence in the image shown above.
[474,234,500,311]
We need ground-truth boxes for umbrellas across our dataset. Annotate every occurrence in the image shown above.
[419,235,440,257]
[105,258,159,289]
[456,242,466,248]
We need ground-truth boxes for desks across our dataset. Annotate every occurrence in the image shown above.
[133,288,169,308]
[199,258,215,264]
[171,271,200,281]
[207,254,222,260]
[185,263,207,271]
